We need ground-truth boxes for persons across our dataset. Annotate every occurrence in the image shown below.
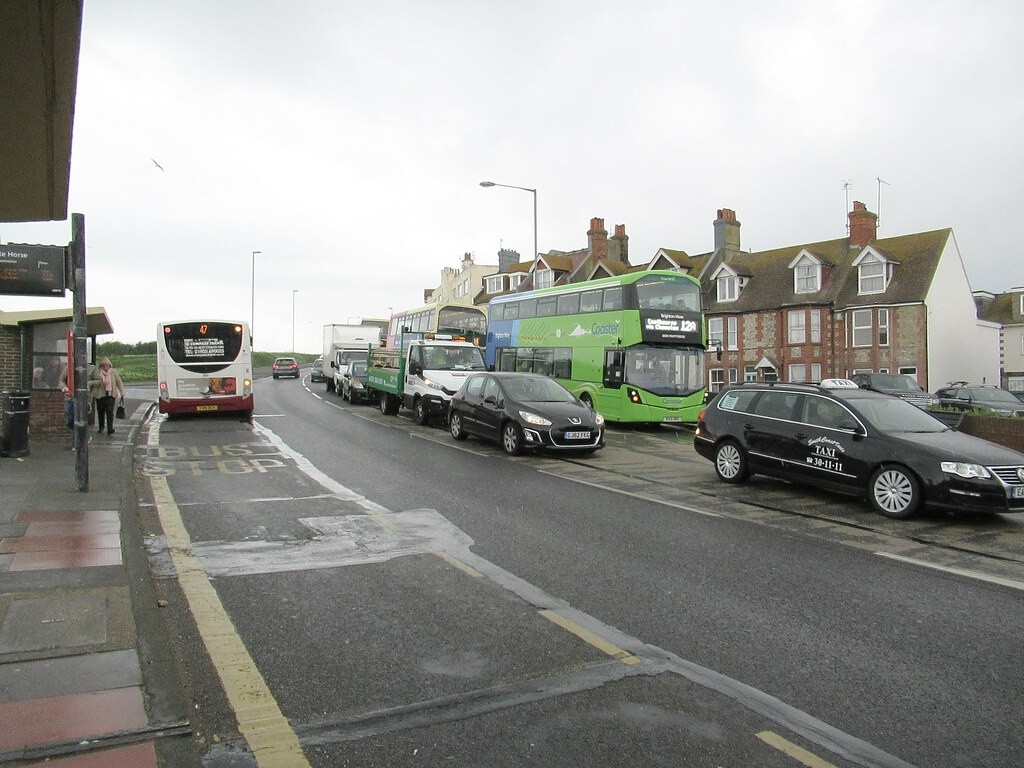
[33,357,63,389]
[59,356,127,451]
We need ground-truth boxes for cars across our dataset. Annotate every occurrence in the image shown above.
[691,375,1024,522]
[342,359,382,406]
[308,359,327,383]
[445,369,607,457]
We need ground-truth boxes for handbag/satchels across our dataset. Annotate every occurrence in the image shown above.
[117,400,125,419]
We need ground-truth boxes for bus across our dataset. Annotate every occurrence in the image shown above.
[484,269,725,425]
[360,318,390,347]
[156,319,255,418]
[384,301,489,369]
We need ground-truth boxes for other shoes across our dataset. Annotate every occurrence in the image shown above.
[108,428,115,435]
[98,427,104,433]
[87,437,92,445]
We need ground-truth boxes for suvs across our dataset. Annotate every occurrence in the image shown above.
[933,381,1024,419]
[271,356,301,380]
[851,372,940,411]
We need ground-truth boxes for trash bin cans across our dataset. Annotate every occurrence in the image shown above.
[2,389,30,458]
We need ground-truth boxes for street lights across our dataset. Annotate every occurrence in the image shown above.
[346,316,362,324]
[478,180,538,289]
[292,288,300,356]
[251,251,263,375]
[388,306,394,315]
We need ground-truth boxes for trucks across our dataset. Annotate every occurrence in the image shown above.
[321,323,382,394]
[364,324,498,426]
